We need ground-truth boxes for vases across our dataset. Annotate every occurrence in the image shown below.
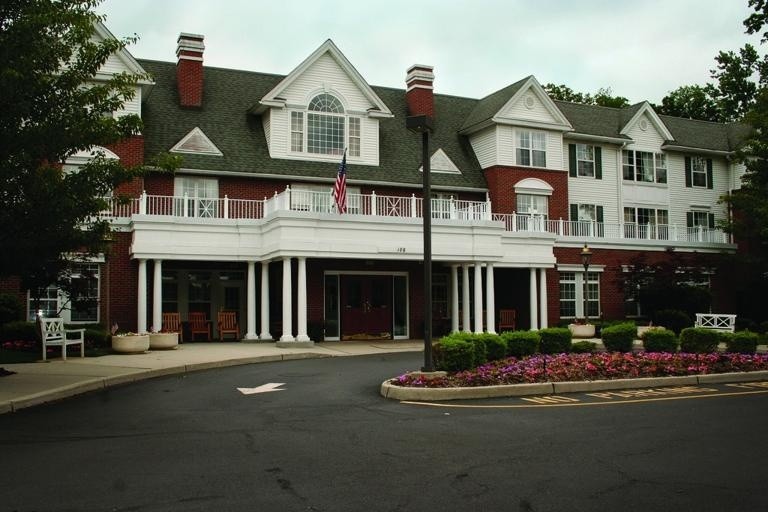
[568,323,596,338]
[111,335,150,353]
[145,333,180,349]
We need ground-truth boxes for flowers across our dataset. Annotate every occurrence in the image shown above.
[115,331,146,337]
[148,325,173,334]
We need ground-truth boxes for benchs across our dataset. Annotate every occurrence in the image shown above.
[694,313,737,333]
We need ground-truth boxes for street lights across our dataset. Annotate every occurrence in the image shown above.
[577,242,593,324]
[406,115,436,372]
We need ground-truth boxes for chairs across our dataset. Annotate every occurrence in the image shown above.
[161,310,240,343]
[499,310,517,332]
[571,316,591,325]
[39,317,87,360]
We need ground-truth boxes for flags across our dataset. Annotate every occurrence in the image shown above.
[330,148,349,215]
